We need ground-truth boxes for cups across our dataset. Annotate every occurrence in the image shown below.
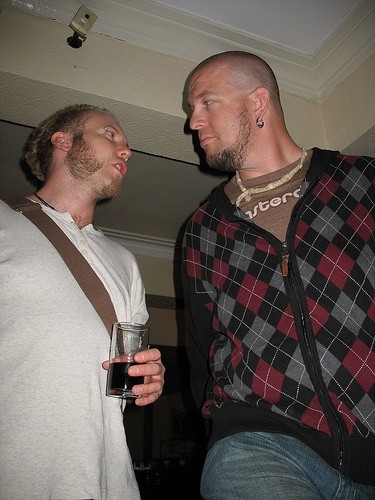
[106,322,151,398]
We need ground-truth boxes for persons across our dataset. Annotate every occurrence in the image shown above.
[178,51,375,500]
[0,104,166,500]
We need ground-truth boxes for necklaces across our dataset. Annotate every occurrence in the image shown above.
[33,192,57,212]
[236,145,307,207]
